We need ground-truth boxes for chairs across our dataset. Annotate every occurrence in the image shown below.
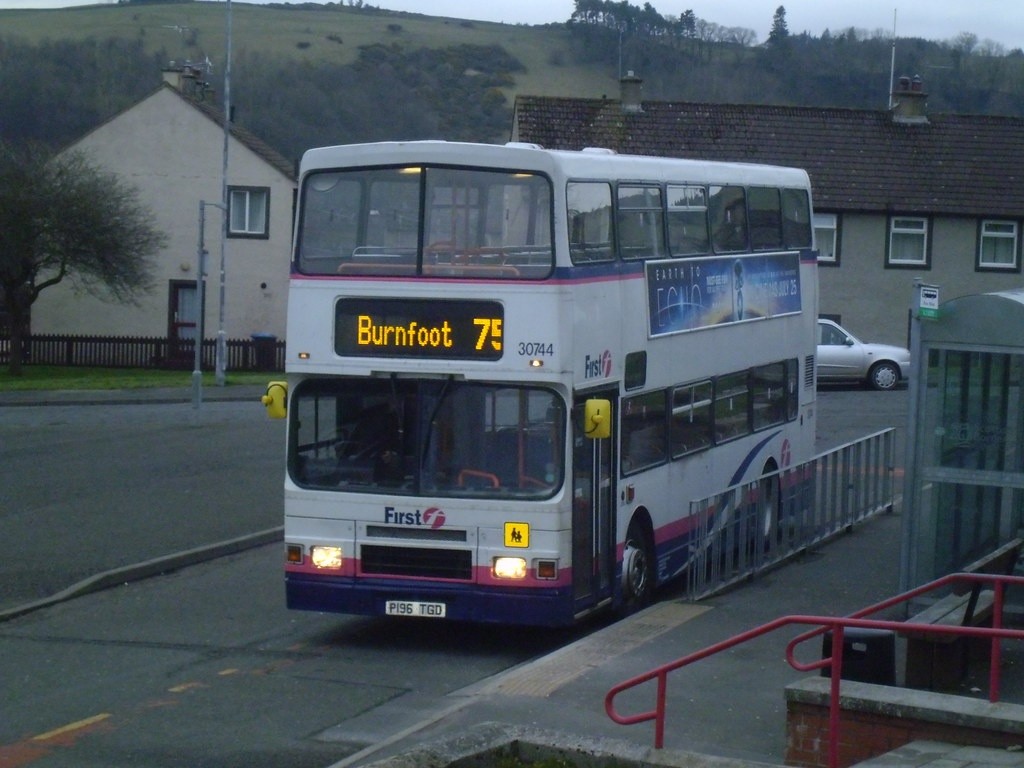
[451,249,510,278]
[412,241,455,275]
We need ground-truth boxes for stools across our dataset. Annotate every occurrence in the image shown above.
[504,241,648,264]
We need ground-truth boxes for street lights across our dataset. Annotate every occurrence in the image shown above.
[190,199,227,430]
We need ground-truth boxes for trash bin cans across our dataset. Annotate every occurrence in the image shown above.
[252,334,276,371]
[820,628,897,686]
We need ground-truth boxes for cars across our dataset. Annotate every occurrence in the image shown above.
[816,318,912,392]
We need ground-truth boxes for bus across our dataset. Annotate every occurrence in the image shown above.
[263,139,821,642]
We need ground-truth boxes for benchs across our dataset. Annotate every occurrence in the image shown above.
[820,538,1024,691]
